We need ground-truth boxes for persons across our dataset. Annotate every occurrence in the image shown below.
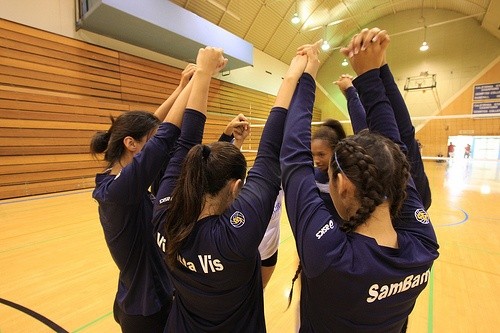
[151,113,284,288]
[92,56,229,333]
[311,73,369,226]
[280,28,440,333]
[151,39,323,333]
[336,27,432,211]
[447,142,472,158]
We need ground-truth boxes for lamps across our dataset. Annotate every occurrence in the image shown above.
[321,25,330,50]
[292,0,300,24]
[420,25,430,52]
